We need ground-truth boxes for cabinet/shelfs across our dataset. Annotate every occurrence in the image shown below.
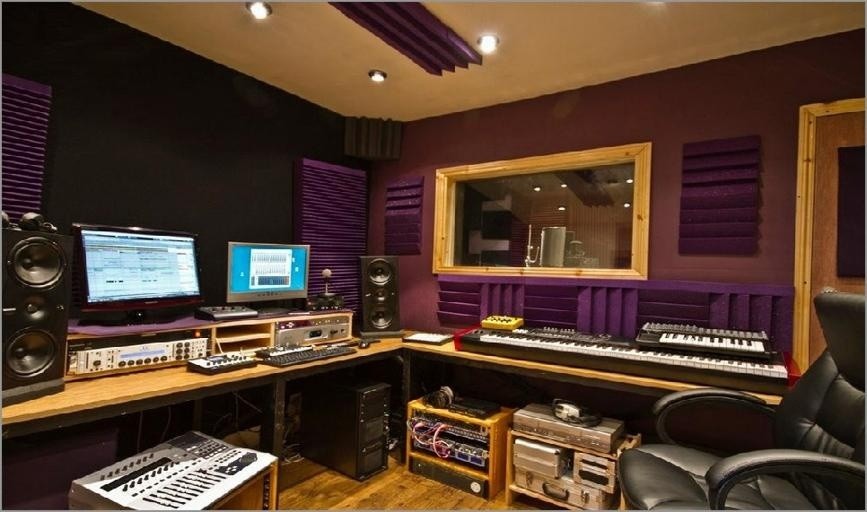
[405,396,521,503]
[503,430,643,512]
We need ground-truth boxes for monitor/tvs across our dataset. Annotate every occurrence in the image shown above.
[226,241,311,317]
[72,222,206,324]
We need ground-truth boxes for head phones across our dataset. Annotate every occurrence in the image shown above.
[432,385,454,408]
[552,397,601,426]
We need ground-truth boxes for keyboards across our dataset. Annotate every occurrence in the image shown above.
[264,347,355,367]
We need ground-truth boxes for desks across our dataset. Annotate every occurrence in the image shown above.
[405,329,801,406]
[1,327,405,498]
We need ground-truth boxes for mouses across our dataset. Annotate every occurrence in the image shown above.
[359,341,368,348]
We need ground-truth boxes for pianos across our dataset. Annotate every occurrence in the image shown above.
[635,322,773,362]
[454,324,791,399]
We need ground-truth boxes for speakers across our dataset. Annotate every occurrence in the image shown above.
[359,256,400,332]
[2,228,73,410]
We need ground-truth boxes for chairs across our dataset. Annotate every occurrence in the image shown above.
[615,292,865,511]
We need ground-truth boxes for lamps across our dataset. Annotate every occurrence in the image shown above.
[626,178,633,184]
[368,69,389,83]
[243,2,274,19]
[476,35,501,54]
[623,202,631,208]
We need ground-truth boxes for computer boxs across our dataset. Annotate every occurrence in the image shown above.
[300,377,391,482]
